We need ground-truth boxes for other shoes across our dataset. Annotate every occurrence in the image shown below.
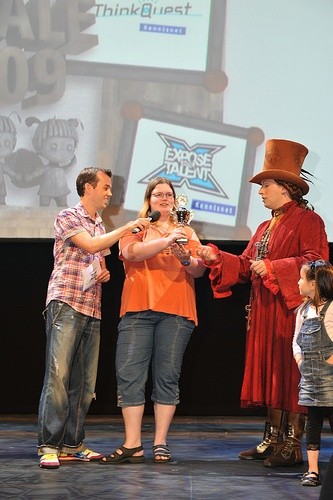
[301,472,321,486]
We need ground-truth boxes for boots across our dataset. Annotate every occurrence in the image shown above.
[239,408,289,459]
[264,412,305,467]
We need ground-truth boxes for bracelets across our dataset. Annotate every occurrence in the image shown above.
[182,259,191,266]
[296,357,303,363]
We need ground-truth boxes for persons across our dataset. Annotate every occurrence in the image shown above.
[196,139,328,467]
[292,260,333,485]
[98,178,204,464]
[37,167,151,470]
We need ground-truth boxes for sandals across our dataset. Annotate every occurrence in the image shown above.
[98,445,145,464]
[152,444,171,463]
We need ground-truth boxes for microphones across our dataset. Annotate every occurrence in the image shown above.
[132,210,161,234]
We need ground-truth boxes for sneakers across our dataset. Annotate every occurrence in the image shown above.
[38,447,60,469]
[58,449,104,462]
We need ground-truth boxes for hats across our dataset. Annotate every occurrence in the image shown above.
[249,139,309,195]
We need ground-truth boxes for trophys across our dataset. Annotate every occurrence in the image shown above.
[170,193,194,242]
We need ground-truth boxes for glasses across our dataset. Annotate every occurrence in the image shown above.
[150,192,174,198]
